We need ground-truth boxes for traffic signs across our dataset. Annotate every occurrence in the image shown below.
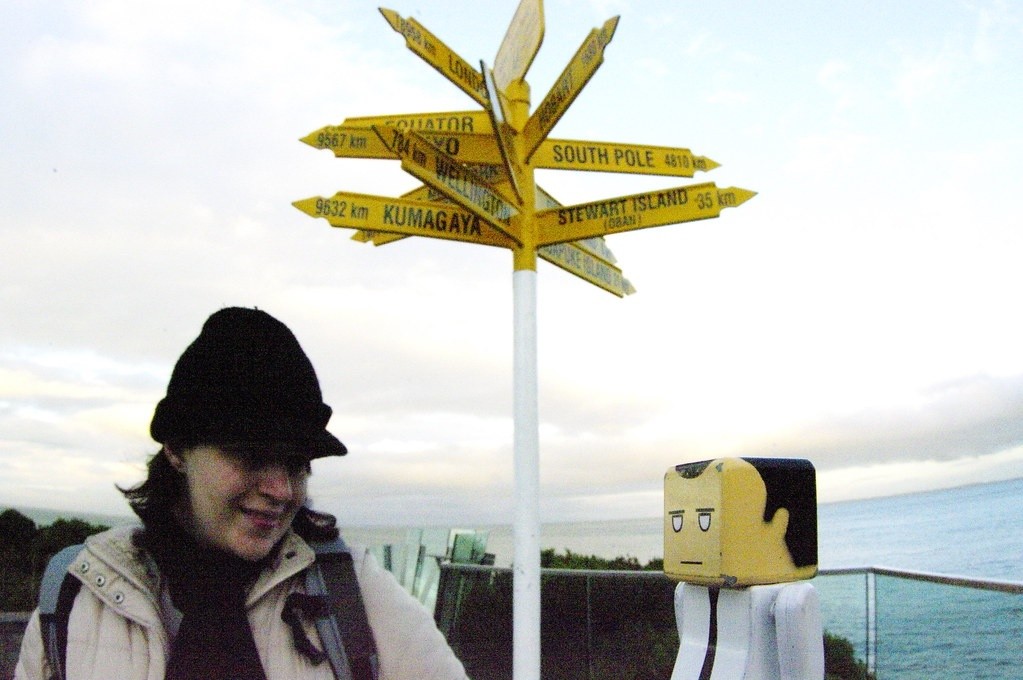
[291,1,759,299]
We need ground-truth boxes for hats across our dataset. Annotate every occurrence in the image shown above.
[150,307,348,457]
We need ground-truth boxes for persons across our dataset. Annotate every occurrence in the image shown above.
[13,306,474,680]
